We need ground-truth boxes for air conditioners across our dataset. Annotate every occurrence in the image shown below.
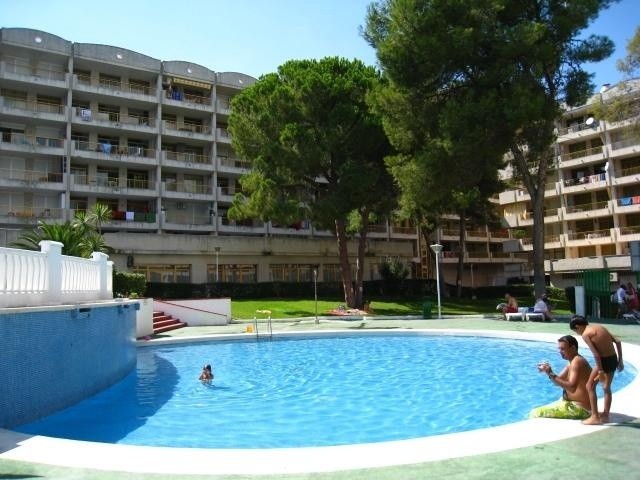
[609,273,618,282]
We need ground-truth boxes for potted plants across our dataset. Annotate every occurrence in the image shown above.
[117,272,154,337]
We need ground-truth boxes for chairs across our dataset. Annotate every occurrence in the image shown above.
[505,307,528,321]
[526,306,552,321]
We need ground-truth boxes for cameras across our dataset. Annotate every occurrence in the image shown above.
[536,361,550,373]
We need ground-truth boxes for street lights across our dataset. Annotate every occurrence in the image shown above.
[430,243,444,319]
[215,246,221,282]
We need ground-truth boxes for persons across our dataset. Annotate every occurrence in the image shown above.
[527,333,595,420]
[497,293,518,321]
[569,314,624,426]
[615,284,627,320]
[627,282,639,308]
[532,296,558,322]
[198,364,215,380]
